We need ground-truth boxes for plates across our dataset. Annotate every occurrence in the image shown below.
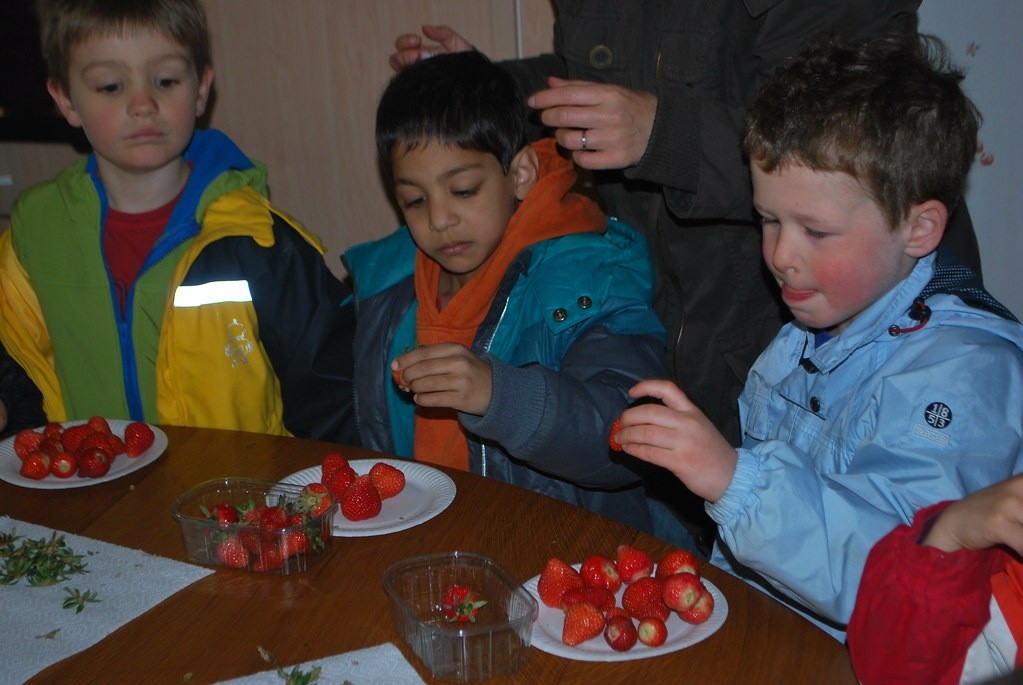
[264,459,456,538]
[509,559,728,663]
[0,419,169,489]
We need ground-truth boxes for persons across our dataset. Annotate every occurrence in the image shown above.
[0,0,362,450]
[346,48,705,557]
[617,28,1022,647]
[386,0,987,552]
[847,474,1023,685]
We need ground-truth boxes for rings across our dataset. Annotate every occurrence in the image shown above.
[582,129,587,149]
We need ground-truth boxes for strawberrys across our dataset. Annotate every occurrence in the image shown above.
[12,416,155,482]
[197,345,718,652]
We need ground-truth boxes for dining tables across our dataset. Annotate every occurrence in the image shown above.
[0,422,862,685]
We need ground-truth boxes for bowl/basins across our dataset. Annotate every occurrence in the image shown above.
[381,550,539,683]
[170,476,339,575]
